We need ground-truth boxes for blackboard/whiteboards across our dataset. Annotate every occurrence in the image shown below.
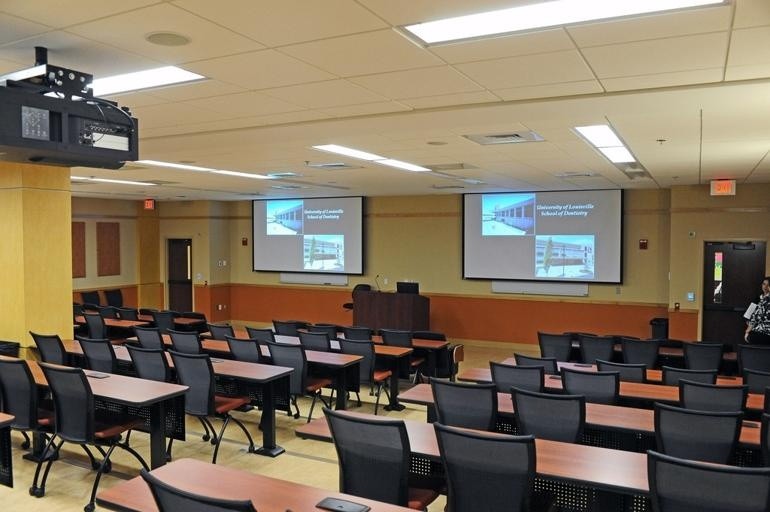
[280,272,348,286]
[491,280,589,297]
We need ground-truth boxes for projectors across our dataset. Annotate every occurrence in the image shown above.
[0,85,138,169]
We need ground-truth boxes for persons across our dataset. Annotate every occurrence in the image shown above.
[744,276,770,346]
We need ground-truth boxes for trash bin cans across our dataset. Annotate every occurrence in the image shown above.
[649,318,669,340]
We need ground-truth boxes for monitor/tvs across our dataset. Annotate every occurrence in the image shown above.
[397,282,419,295]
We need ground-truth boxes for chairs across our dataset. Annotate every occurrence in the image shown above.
[389,323,770,511]
[319,400,437,512]
[80,289,103,306]
[343,285,375,311]
[136,463,254,511]
[102,285,124,306]
[1,355,102,495]
[34,356,152,512]
[26,300,452,458]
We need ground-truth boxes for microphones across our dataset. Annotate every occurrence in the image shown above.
[375,275,381,292]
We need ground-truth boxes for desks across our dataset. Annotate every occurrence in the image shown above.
[1,350,190,470]
[1,409,20,493]
[90,455,423,512]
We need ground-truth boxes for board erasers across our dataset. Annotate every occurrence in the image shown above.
[324,283,331,286]
